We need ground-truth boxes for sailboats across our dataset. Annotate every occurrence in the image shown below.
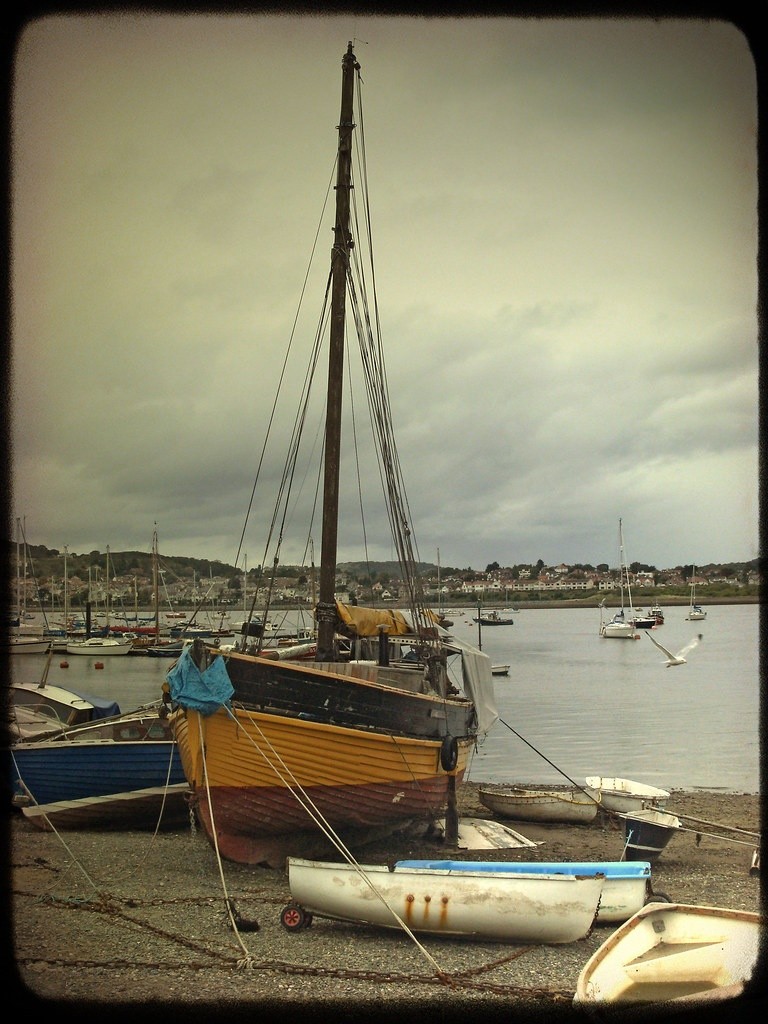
[157,40,481,864]
[7,515,315,656]
[600,518,636,639]
[688,563,707,621]
[436,545,465,617]
[477,599,511,676]
[503,588,519,613]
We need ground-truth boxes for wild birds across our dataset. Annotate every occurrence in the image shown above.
[644,630,703,668]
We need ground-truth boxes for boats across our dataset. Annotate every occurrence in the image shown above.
[5,636,123,744]
[285,855,608,947]
[627,607,657,630]
[476,787,602,825]
[586,774,672,816]
[8,698,195,832]
[571,902,764,1003]
[472,611,513,625]
[647,602,666,625]
[397,859,653,923]
